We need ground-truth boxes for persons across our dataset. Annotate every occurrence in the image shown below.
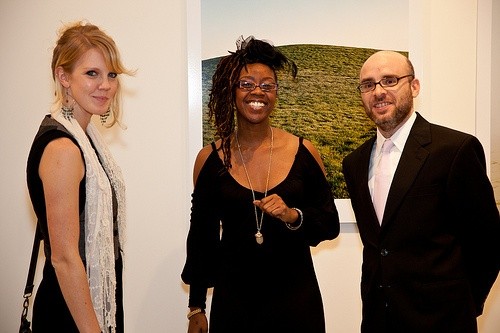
[342,50,500,333]
[181,36,340,333]
[26,23,137,333]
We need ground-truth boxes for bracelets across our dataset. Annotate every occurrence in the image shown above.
[285,208,304,230]
[187,308,207,319]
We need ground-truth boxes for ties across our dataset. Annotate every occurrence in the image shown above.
[372,140,394,227]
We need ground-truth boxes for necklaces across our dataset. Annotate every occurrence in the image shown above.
[234,127,274,244]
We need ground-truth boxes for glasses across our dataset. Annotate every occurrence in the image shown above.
[236,80,279,94]
[357,74,414,93]
